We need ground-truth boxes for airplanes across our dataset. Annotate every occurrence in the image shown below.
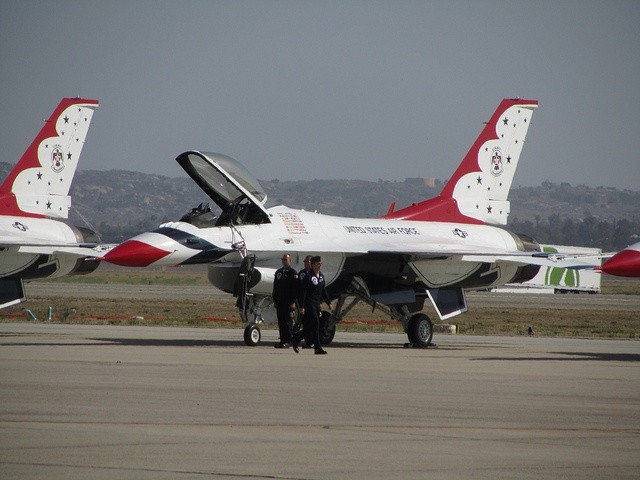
[0,97,120,310]
[592,242,640,277]
[84,96,620,348]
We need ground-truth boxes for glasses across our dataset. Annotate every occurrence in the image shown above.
[304,260,309,262]
[281,258,287,261]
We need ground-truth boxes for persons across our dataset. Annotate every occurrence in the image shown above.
[272,253,299,348]
[299,255,313,346]
[292,256,331,354]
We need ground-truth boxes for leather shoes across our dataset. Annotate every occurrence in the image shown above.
[291,341,299,353]
[275,343,289,348]
[302,344,315,348]
[314,347,327,354]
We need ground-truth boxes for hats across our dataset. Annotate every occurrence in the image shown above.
[311,256,322,263]
